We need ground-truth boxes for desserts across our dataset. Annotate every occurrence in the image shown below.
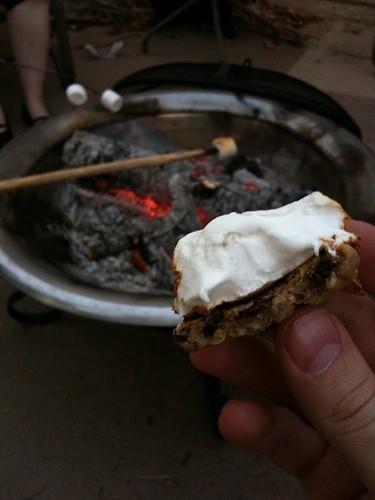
[170,190,362,352]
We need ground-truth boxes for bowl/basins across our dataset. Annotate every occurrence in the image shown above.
[0,87,371,325]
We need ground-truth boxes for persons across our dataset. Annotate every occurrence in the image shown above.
[0,0,77,153]
[186,216,375,500]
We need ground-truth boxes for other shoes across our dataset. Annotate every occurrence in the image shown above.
[1,110,12,147]
[23,101,52,126]
